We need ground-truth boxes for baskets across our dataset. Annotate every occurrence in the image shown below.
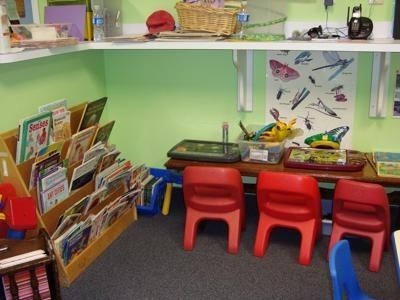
[174,1,247,36]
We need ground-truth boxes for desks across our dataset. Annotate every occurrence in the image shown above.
[392,230,400,284]
[164,152,399,204]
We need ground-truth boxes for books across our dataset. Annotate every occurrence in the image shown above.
[10,92,167,266]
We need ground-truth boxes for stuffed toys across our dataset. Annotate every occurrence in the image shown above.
[238,109,303,166]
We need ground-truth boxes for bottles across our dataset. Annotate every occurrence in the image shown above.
[92,5,104,42]
[235,1,250,41]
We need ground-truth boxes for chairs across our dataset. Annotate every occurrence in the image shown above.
[326,178,390,272]
[183,165,246,254]
[329,240,372,300]
[252,171,322,266]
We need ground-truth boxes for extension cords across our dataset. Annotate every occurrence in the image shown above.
[311,34,339,41]
